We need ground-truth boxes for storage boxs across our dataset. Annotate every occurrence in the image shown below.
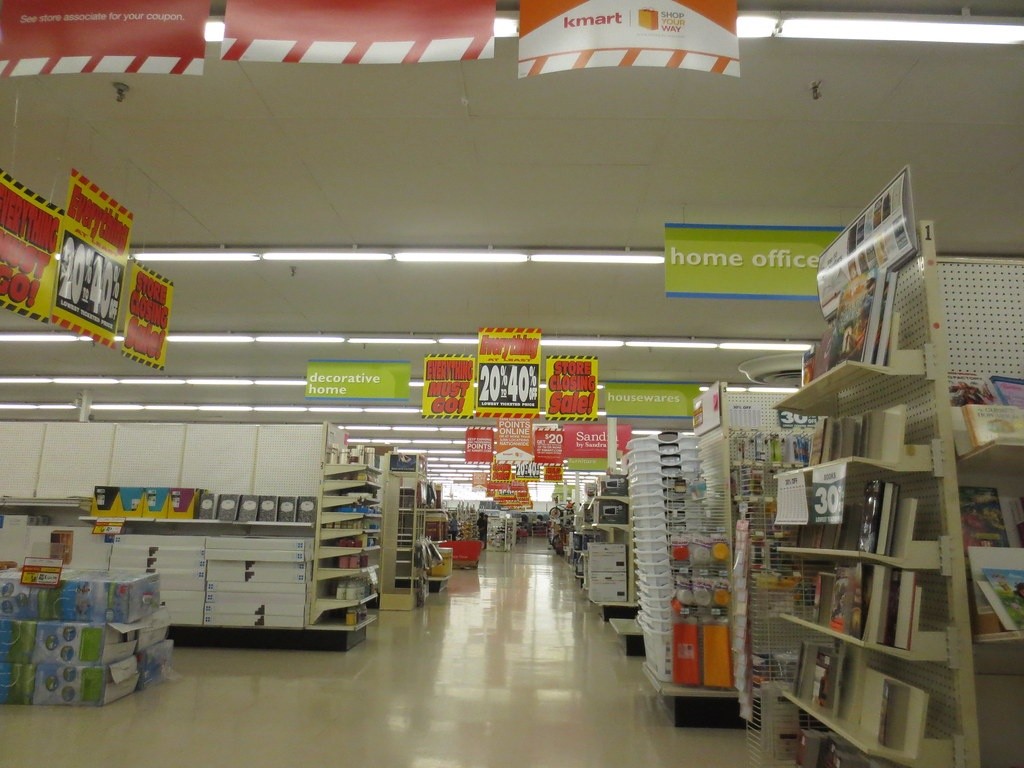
[1,486,314,706]
[587,543,627,601]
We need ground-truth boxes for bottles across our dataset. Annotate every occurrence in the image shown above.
[330,443,375,467]
[336,553,368,626]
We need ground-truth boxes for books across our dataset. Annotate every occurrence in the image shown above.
[801,264,897,386]
[815,562,915,649]
[809,413,871,464]
[798,730,893,768]
[958,486,1024,633]
[810,639,896,746]
[947,372,1024,452]
[813,479,898,555]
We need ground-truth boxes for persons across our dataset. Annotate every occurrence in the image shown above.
[448,512,457,540]
[476,512,488,549]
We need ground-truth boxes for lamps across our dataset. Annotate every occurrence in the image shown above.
[3,12,1022,484]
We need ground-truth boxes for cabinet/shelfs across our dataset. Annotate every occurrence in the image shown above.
[381,471,429,610]
[312,464,384,652]
[630,216,1023,768]
[426,508,583,579]
[579,495,642,622]
[0,496,310,649]
